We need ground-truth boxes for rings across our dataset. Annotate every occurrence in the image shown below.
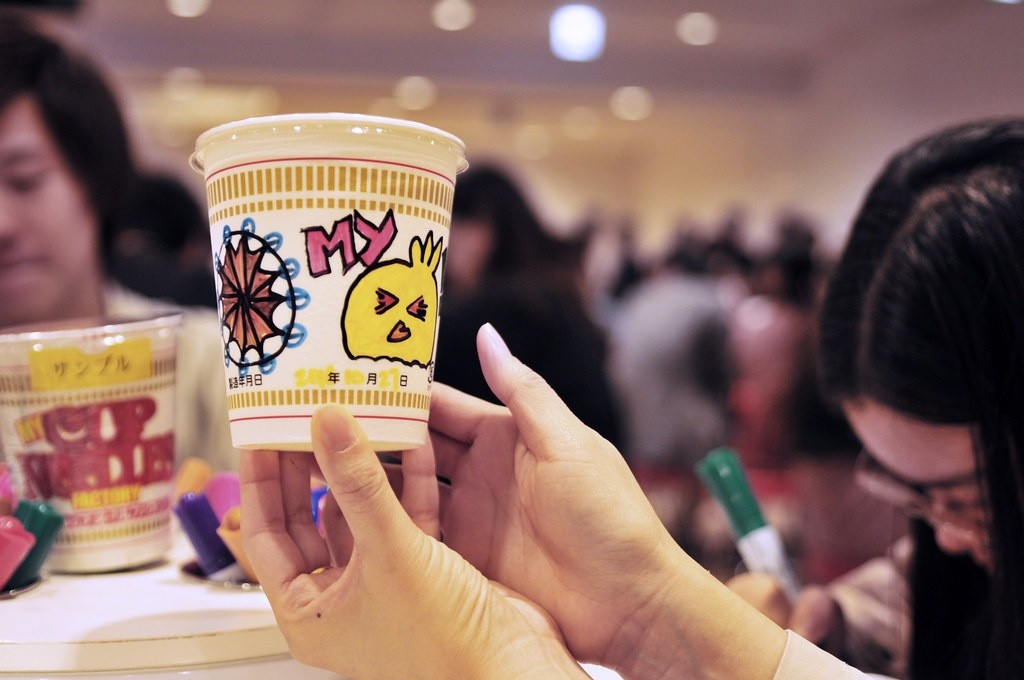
[310,565,334,574]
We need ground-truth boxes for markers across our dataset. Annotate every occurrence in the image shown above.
[701,445,805,602]
[172,474,327,591]
[0,500,61,593]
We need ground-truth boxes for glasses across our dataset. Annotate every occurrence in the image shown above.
[851,446,996,534]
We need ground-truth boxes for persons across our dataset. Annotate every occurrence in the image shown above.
[433,163,907,580]
[235,322,897,680]
[0,21,234,500]
[699,118,1024,680]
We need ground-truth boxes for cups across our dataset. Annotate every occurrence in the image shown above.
[0,308,185,574]
[189,110,471,454]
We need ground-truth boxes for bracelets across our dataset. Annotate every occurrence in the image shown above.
[820,583,846,640]
